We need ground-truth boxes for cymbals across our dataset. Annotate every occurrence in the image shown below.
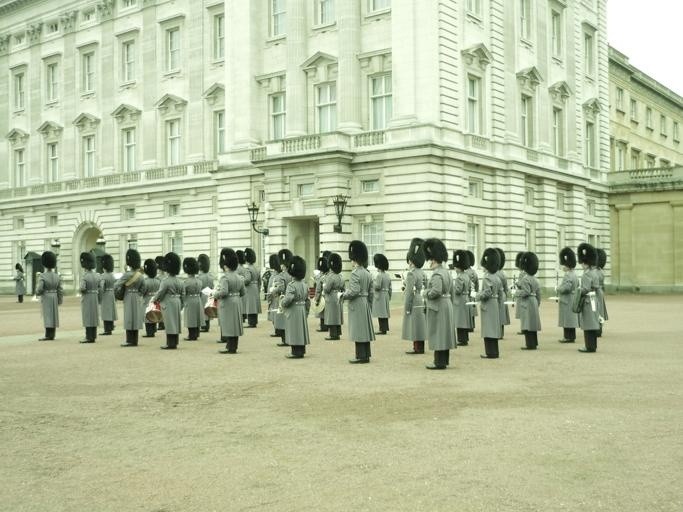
[311,295,326,315]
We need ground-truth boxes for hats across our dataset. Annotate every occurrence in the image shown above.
[16,264,20,269]
[269,249,306,279]
[318,240,388,279]
[406,237,539,275]
[560,243,606,268]
[41,251,56,269]
[80,249,256,275]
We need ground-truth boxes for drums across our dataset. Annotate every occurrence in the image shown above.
[203,296,217,318]
[145,300,162,323]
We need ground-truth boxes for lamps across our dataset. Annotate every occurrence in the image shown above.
[332,193,352,232]
[246,201,268,236]
[96,234,106,253]
[51,237,61,257]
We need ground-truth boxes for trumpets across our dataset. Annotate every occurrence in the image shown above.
[276,292,285,314]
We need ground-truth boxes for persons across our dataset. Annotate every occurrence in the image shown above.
[34,251,64,341]
[312,239,393,364]
[75,244,311,358]
[397,237,542,369]
[554,243,608,354]
[12,262,28,303]
